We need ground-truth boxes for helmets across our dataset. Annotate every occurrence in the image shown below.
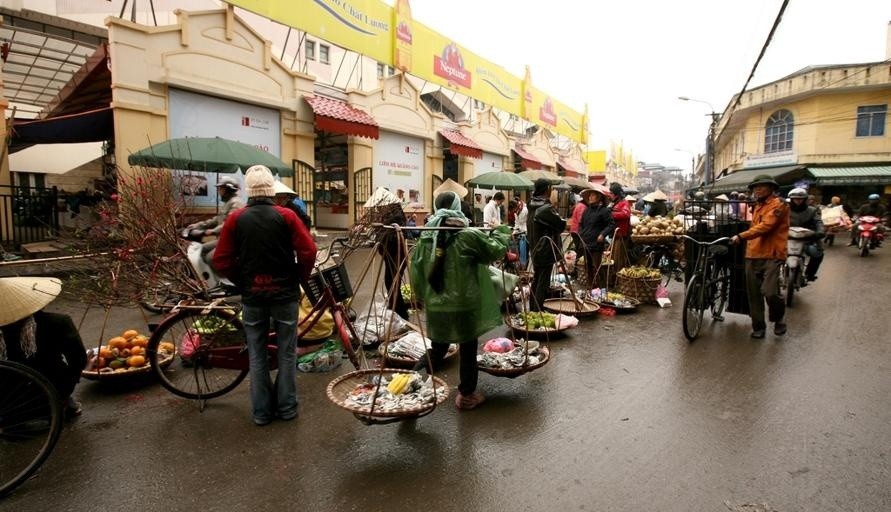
[788,188,808,199]
[868,194,880,203]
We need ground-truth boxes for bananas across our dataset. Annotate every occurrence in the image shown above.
[387,373,412,396]
[620,266,660,278]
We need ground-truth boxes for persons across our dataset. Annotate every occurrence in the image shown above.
[846,193,885,247]
[807,194,822,219]
[406,212,421,240]
[187,175,244,264]
[363,186,410,322]
[728,173,790,340]
[275,178,312,231]
[212,164,318,425]
[624,189,753,224]
[570,200,589,286]
[0,277,89,422]
[787,188,826,281]
[606,182,631,238]
[507,197,529,272]
[409,192,512,409]
[526,177,567,312]
[822,196,851,247]
[579,188,617,283]
[482,191,506,237]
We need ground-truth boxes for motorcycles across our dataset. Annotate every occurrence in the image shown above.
[850,216,888,258]
[135,226,219,313]
[779,226,824,308]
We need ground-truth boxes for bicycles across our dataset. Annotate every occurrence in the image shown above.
[0,359,64,499]
[675,234,734,342]
[145,236,371,414]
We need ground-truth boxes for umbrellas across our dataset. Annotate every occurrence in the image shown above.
[127,137,294,216]
[468,170,535,192]
[517,169,566,185]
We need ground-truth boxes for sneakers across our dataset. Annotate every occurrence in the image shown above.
[751,329,765,339]
[774,320,786,335]
[455,390,485,410]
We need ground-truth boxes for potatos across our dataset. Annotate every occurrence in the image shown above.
[632,217,685,237]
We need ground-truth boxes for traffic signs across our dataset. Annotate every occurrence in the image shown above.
[678,95,716,185]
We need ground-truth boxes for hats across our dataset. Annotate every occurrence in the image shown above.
[579,188,606,199]
[747,174,779,189]
[714,194,729,201]
[214,177,239,190]
[273,179,296,195]
[433,178,468,199]
[0,276,62,327]
[244,165,276,198]
[364,187,401,207]
[625,195,636,201]
[642,192,654,202]
[654,190,667,200]
